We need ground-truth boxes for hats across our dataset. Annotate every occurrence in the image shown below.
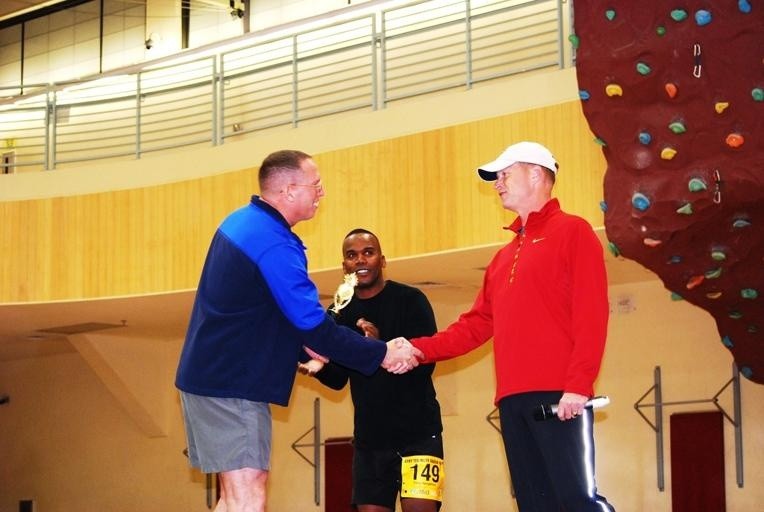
[477,141,558,181]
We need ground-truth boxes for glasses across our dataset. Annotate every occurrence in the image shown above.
[292,180,322,189]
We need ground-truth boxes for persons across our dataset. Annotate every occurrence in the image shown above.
[296,228,446,512]
[379,140,616,512]
[174,149,426,512]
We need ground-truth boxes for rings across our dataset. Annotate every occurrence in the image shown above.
[570,410,577,415]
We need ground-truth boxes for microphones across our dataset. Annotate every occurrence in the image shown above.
[533,395,610,421]
